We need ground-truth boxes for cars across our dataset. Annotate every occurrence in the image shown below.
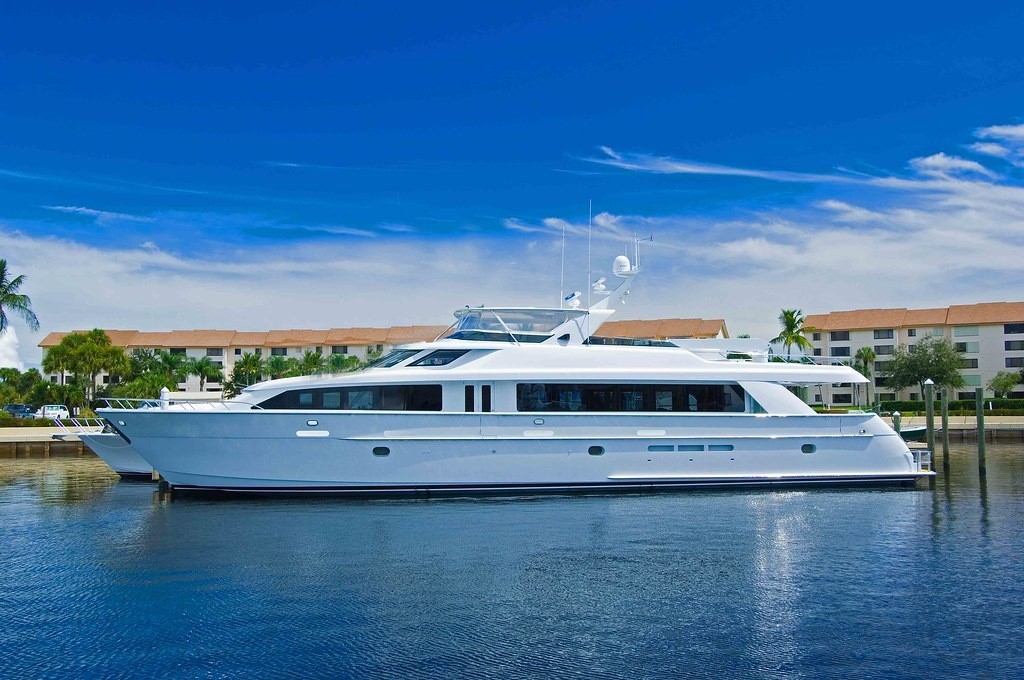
[35,405,70,420]
[2,404,35,420]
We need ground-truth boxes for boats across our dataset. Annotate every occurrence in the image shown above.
[96,198,936,496]
[52,415,163,479]
